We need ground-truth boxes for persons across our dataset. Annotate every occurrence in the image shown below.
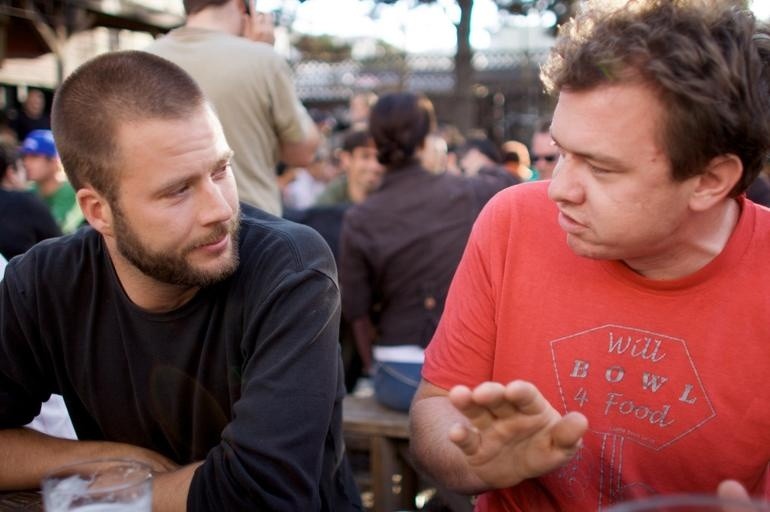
[142,0,561,365]
[0,90,88,261]
[407,0,768,511]
[2,50,361,511]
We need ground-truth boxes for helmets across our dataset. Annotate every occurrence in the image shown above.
[22,130,57,156]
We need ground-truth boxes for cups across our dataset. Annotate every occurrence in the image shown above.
[40,460,153,511]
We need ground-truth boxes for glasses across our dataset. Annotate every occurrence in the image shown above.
[529,155,554,162]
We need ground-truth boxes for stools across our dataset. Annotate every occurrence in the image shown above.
[335,394,420,512]
